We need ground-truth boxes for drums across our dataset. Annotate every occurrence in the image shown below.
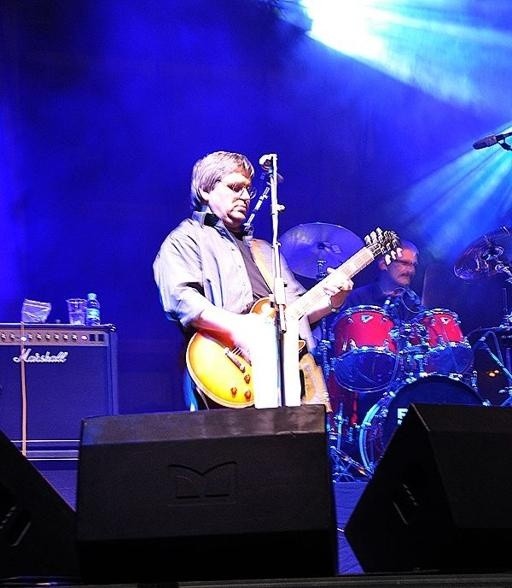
[404,307,473,375]
[360,373,484,474]
[318,367,358,440]
[330,303,404,394]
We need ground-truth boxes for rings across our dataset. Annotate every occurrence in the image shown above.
[337,285,343,289]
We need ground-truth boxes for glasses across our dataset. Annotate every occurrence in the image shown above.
[228,182,258,199]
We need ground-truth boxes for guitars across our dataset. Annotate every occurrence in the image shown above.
[186,227,403,407]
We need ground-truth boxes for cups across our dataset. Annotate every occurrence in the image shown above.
[65,299,87,325]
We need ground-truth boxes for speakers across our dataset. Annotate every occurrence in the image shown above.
[0,429,76,587]
[345,401,496,563]
[77,405,337,576]
[0,344,116,460]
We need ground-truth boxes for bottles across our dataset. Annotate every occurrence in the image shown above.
[86,292,100,326]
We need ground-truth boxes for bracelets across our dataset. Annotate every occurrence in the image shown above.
[326,299,344,312]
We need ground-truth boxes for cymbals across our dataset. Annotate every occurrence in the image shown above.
[279,222,363,279]
[455,227,510,279]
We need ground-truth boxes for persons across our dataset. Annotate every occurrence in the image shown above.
[342,237,423,324]
[151,149,355,409]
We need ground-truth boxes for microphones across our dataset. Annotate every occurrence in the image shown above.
[474,131,512,151]
[259,154,276,173]
